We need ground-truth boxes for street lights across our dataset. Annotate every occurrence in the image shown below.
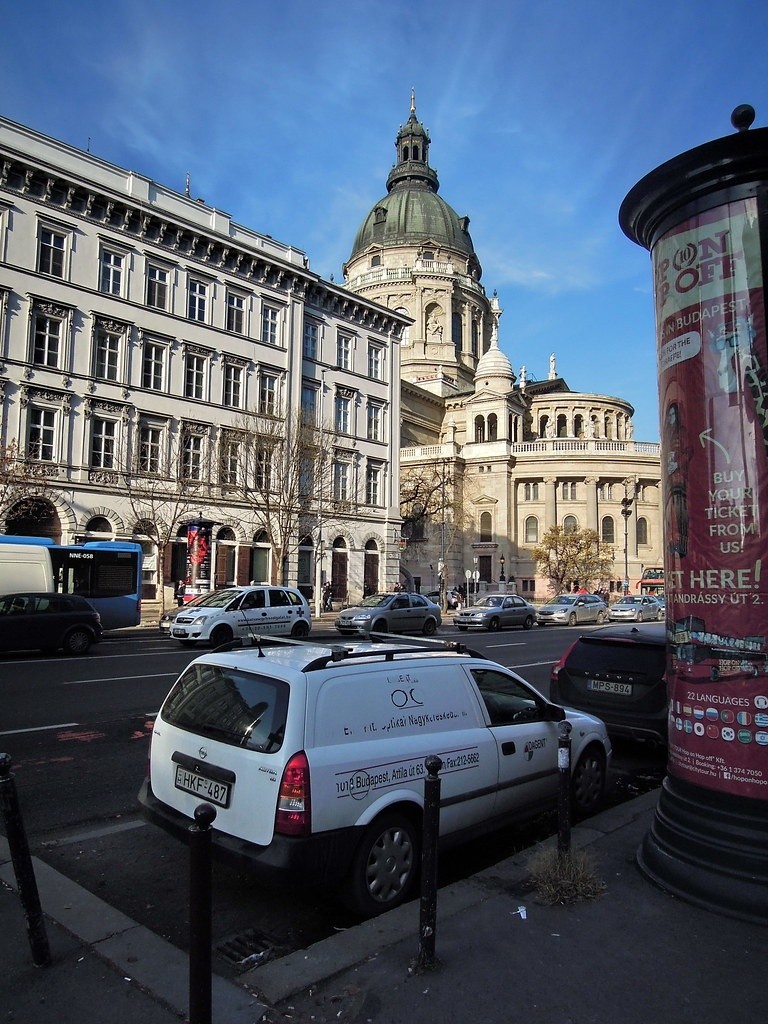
[315,365,342,617]
[474,555,479,603]
[441,457,453,614]
[621,475,634,594]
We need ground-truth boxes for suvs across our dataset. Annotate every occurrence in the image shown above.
[134,630,616,917]
[549,617,667,778]
[422,590,461,608]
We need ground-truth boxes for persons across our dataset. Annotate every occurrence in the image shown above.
[550,353,556,373]
[322,581,333,612]
[74,579,88,592]
[363,583,370,599]
[176,579,186,606]
[7,599,25,615]
[453,585,467,598]
[598,590,609,607]
[400,584,406,591]
[457,593,462,610]
[394,582,400,592]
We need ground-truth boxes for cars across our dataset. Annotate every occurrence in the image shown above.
[535,593,607,625]
[335,590,442,639]
[608,595,660,622]
[657,599,665,617]
[157,586,312,649]
[0,593,105,659]
[453,595,535,632]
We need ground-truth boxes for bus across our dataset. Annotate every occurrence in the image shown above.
[636,567,665,598]
[0,535,143,635]
[671,637,766,682]
